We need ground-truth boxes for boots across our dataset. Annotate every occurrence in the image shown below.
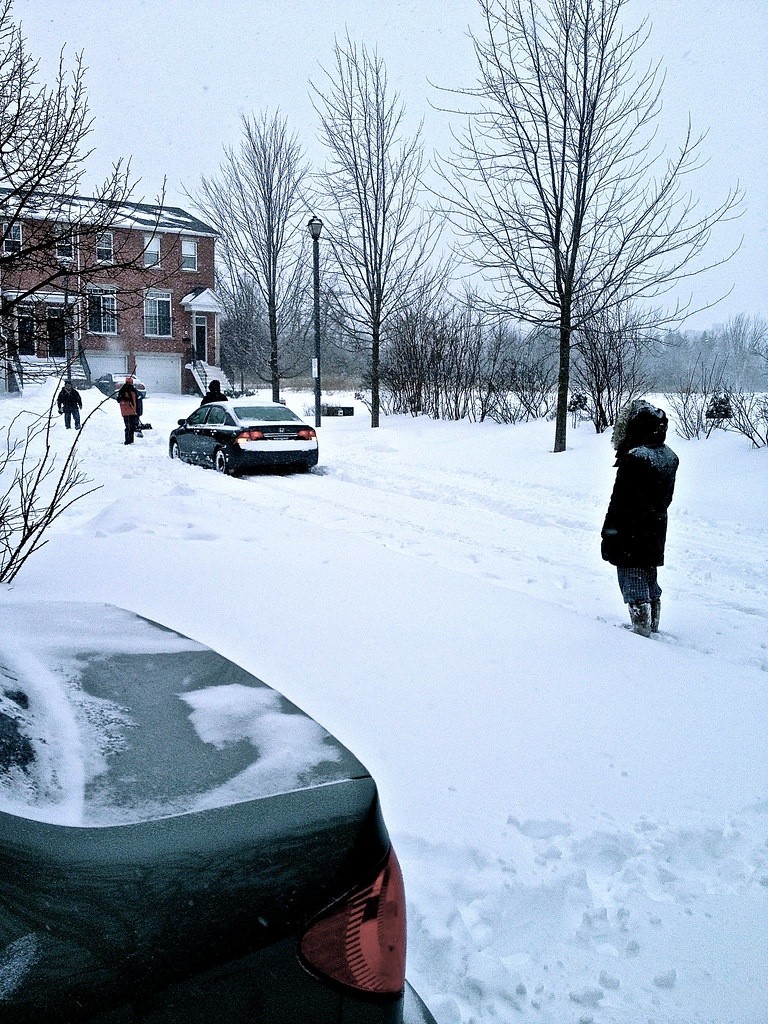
[650,602,661,632]
[620,602,651,637]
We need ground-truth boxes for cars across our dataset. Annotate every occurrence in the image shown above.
[169,403,319,477]
[0,599,441,1024]
[93,372,148,404]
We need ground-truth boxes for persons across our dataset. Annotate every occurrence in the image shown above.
[57,381,83,430]
[600,399,679,638]
[117,378,144,444]
[200,380,228,406]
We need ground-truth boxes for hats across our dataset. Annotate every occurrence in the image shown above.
[126,376,133,384]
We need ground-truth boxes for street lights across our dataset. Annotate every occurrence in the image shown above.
[305,214,325,427]
